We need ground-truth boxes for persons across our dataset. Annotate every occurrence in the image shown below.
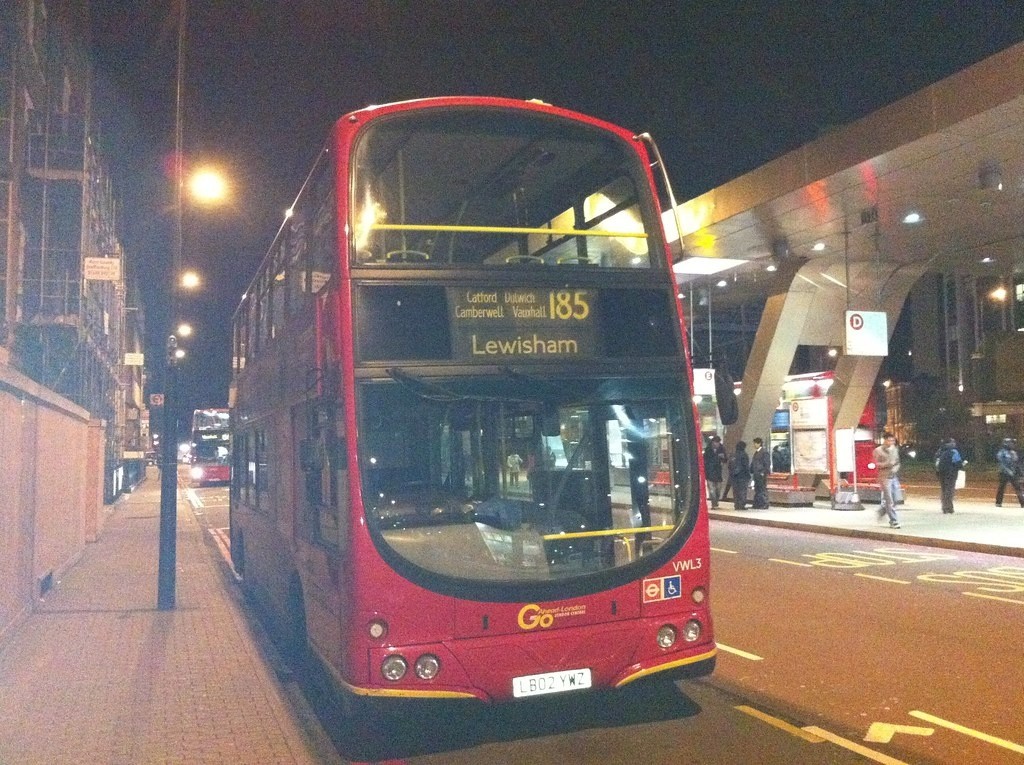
[704,436,727,510]
[507,449,523,488]
[528,446,556,478]
[996,438,1024,508]
[749,437,771,509]
[772,444,790,472]
[873,434,901,529]
[933,438,964,514]
[728,441,751,510]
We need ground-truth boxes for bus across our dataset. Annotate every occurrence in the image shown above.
[227,92,745,715]
[735,370,881,482]
[180,409,230,486]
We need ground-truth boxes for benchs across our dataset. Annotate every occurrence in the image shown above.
[766,484,816,507]
[841,483,907,504]
[648,472,672,495]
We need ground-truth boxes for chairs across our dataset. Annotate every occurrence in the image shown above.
[557,256,599,266]
[387,250,430,262]
[505,256,545,265]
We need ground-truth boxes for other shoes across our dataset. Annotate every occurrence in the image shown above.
[711,504,718,510]
[890,523,900,529]
[943,507,954,513]
[1021,502,1024,508]
[734,505,749,510]
[753,503,769,509]
[995,501,1001,507]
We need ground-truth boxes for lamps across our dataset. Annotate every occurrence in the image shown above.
[979,172,1002,193]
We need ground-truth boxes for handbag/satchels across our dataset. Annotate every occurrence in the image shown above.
[935,458,940,472]
